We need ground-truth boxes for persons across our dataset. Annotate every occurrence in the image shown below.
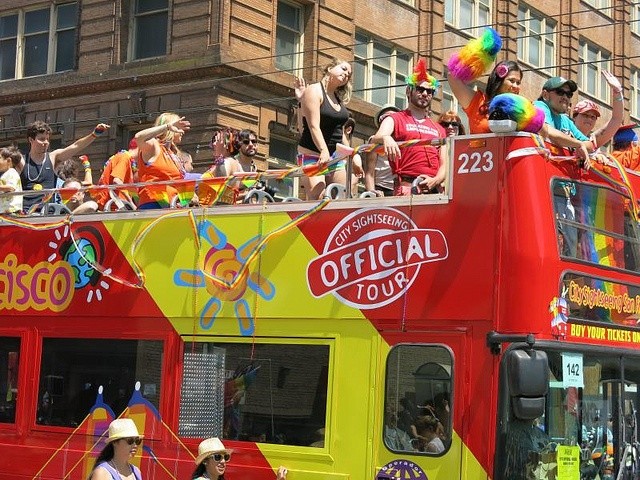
[235,129,258,172]
[533,417,545,432]
[447,28,549,136]
[90,418,142,480]
[365,103,404,197]
[275,366,299,400]
[582,424,601,480]
[54,155,92,216]
[596,412,614,480]
[301,58,365,199]
[373,59,448,194]
[572,70,624,165]
[180,152,204,190]
[383,392,449,455]
[293,76,362,197]
[0,145,23,214]
[15,121,110,215]
[134,112,191,209]
[97,137,141,209]
[62,177,99,216]
[437,112,465,137]
[195,128,246,195]
[533,77,597,171]
[190,438,289,480]
[612,123,640,170]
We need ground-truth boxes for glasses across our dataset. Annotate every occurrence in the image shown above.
[548,89,573,98]
[416,86,435,94]
[207,454,231,462]
[440,121,460,127]
[124,437,142,445]
[238,140,257,144]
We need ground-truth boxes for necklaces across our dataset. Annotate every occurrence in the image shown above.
[203,473,210,480]
[27,152,47,182]
[111,458,136,480]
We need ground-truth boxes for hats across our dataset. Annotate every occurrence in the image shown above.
[543,77,577,92]
[104,418,144,446]
[374,104,401,128]
[573,101,601,117]
[614,128,638,141]
[195,437,232,466]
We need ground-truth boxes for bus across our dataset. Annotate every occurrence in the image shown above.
[0,132,640,480]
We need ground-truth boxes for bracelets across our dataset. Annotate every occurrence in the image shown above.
[613,97,623,102]
[613,96,624,101]
